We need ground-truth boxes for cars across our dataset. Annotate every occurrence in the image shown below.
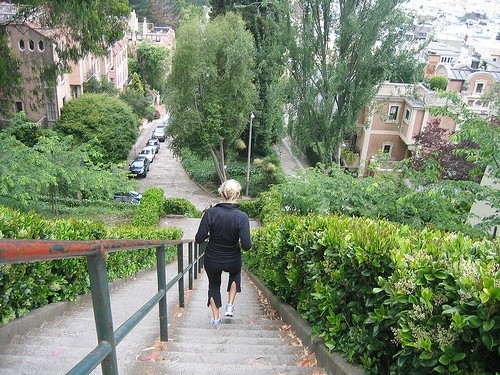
[152,128,167,143]
[129,156,150,178]
[145,138,160,149]
[115,190,142,203]
[138,145,157,163]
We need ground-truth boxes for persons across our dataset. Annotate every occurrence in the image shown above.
[196,179,252,324]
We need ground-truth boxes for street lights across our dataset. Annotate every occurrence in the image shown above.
[245,112,255,196]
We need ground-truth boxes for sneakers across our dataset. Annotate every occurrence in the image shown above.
[224,304,235,317]
[212,318,222,329]
